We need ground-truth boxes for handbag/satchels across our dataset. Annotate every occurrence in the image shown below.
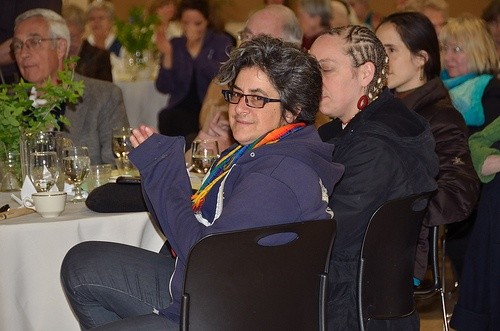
[85,182,201,212]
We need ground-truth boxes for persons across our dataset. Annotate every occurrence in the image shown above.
[1,0,500,331]
[61,35,345,331]
[309,25,438,331]
[11,9,130,165]
[374,12,480,291]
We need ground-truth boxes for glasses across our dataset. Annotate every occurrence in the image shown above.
[440,46,464,56]
[11,38,61,51]
[237,31,255,43]
[221,89,282,108]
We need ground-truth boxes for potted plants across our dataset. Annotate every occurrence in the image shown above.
[115,6,161,70]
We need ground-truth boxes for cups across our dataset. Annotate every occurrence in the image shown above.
[112,127,134,174]
[19,131,59,185]
[29,151,60,193]
[23,192,67,218]
[191,139,219,173]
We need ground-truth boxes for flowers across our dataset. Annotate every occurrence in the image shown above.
[0,53,89,155]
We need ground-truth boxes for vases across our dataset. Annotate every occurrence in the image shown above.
[2,150,23,191]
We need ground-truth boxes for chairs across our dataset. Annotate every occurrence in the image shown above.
[358,191,431,331]
[418,224,450,331]
[179,218,339,331]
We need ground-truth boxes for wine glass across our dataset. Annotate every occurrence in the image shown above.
[61,146,90,203]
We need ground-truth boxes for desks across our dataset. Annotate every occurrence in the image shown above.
[0,164,207,331]
[117,82,169,131]
